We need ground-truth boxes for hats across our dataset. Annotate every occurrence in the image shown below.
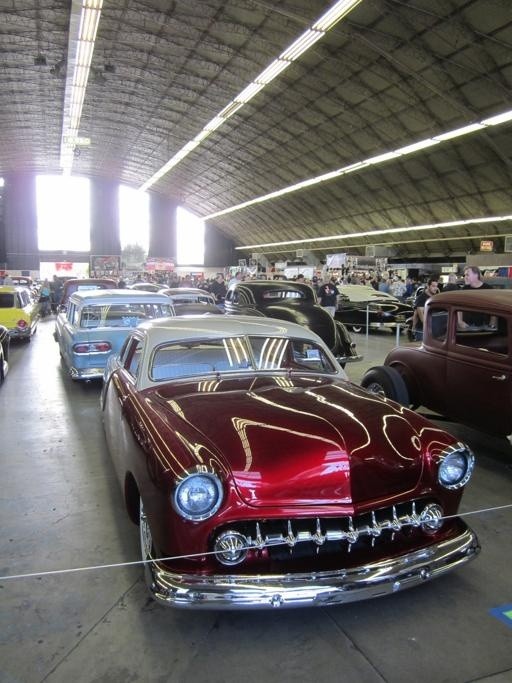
[98,312,484,614]
[360,287,512,454]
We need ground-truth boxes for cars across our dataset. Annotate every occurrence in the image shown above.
[159,287,225,317]
[53,289,176,379]
[219,280,365,375]
[98,312,484,614]
[360,287,512,454]
[1,272,512,343]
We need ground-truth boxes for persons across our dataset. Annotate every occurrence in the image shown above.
[0,274,13,286]
